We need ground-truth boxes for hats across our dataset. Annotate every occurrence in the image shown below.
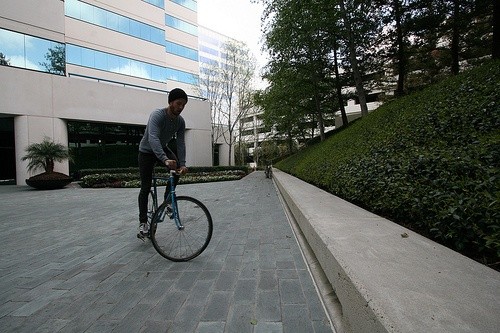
[167,88,188,102]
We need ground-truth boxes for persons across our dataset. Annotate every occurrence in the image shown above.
[136,88,188,239]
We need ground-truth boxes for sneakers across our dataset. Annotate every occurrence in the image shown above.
[139,221,150,236]
[167,203,173,212]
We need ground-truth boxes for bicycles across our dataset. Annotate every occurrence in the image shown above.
[145,162,213,262]
